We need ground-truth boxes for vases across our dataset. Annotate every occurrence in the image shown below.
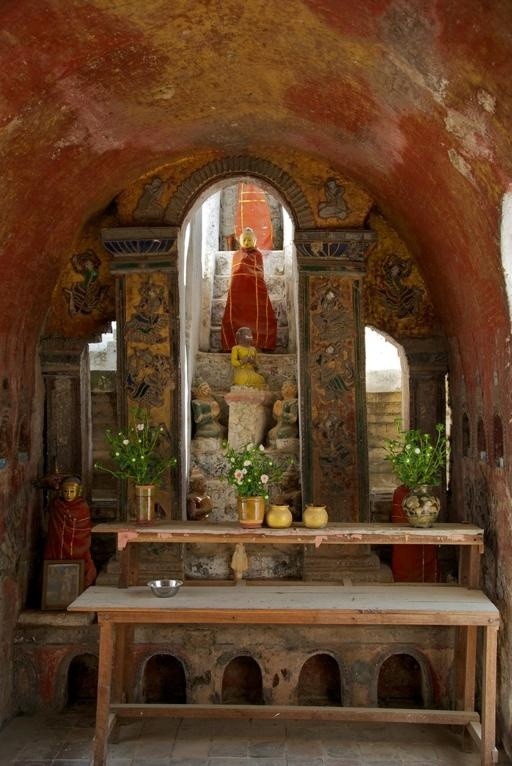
[235,496,266,528]
[400,485,442,530]
[130,485,160,526]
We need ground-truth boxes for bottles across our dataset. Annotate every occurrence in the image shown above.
[267,504,293,529]
[303,503,328,529]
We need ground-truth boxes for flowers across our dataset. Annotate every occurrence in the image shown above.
[380,417,455,489]
[220,443,294,498]
[96,403,180,484]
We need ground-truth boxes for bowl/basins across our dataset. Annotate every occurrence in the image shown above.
[148,579,183,598]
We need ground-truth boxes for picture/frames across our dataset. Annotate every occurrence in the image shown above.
[40,559,85,614]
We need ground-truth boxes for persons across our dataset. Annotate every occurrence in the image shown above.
[187,472,213,520]
[32,473,97,610]
[262,376,300,451]
[315,174,351,220]
[229,325,263,388]
[219,226,278,354]
[132,175,169,225]
[272,471,302,523]
[190,376,224,441]
